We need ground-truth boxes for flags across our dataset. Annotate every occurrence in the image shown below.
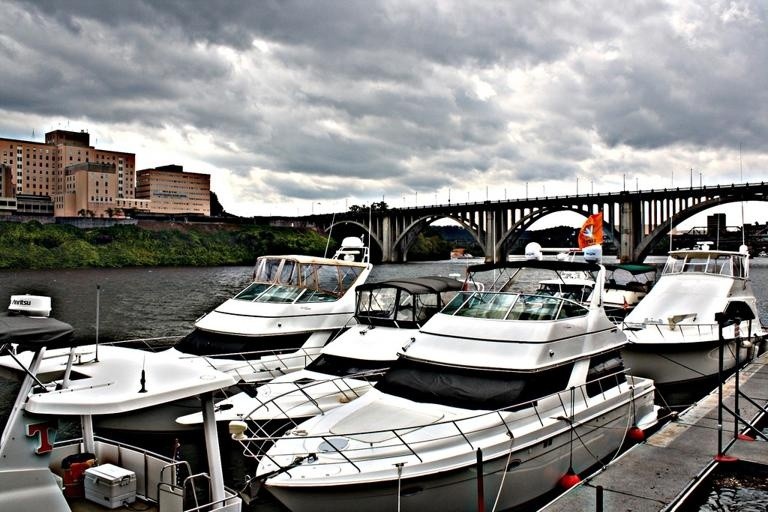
[577,212,603,250]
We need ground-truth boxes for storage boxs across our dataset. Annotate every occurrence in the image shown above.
[80,462,136,509]
